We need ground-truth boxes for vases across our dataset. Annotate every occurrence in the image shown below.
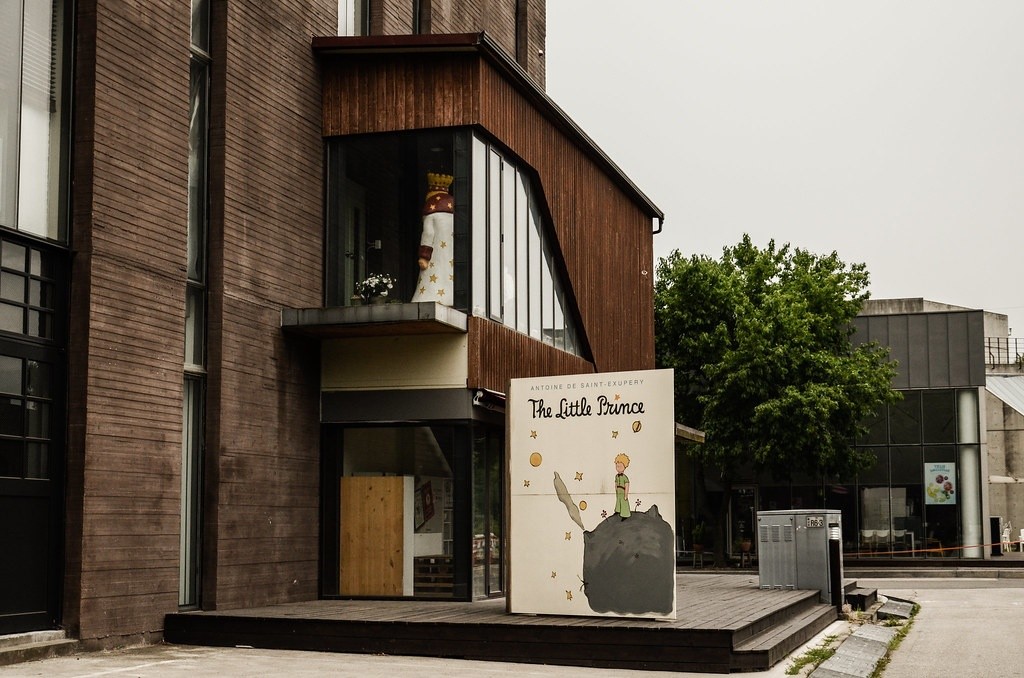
[371,295,387,304]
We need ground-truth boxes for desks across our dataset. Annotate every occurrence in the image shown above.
[692,551,704,569]
[735,551,752,569]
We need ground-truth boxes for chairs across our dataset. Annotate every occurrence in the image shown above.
[858,529,915,557]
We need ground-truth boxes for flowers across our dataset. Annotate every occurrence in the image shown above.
[355,272,397,304]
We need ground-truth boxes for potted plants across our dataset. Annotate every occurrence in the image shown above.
[733,538,751,552]
[691,520,707,552]
